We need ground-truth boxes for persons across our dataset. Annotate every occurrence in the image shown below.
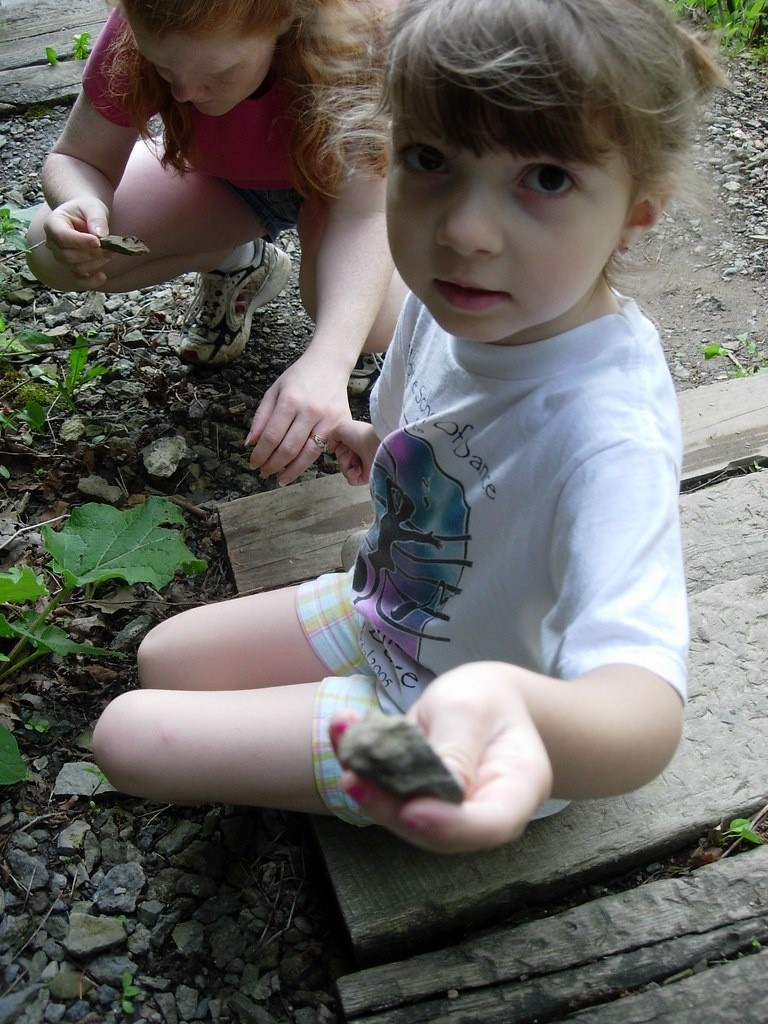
[25,0,412,487]
[90,0,728,851]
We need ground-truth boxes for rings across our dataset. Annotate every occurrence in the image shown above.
[309,433,330,453]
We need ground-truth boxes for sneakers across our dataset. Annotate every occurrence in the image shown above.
[180,238,292,364]
[347,351,386,398]
